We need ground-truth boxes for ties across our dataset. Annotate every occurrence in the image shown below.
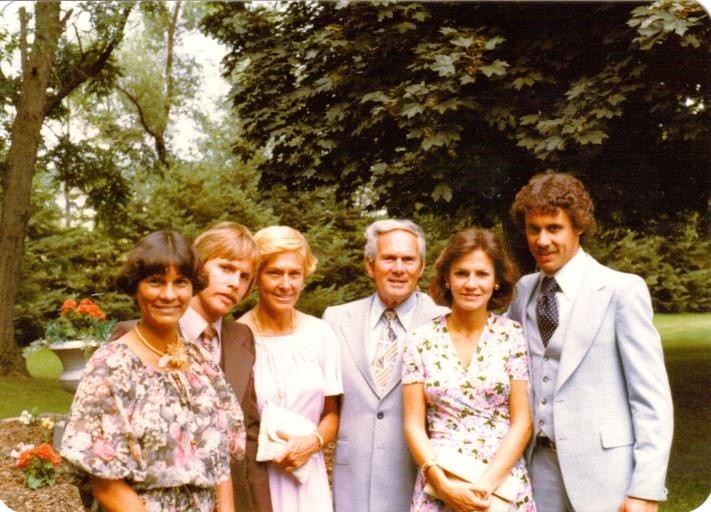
[536,276,558,348]
[370,311,398,392]
[201,332,212,341]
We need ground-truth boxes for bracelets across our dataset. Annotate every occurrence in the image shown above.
[314,432,323,451]
[420,459,437,472]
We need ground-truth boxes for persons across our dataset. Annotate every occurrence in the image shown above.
[61,226,246,512]
[402,228,536,511]
[505,174,673,512]
[236,224,339,511]
[113,223,273,512]
[322,219,452,511]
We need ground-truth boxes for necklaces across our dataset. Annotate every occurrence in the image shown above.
[253,304,295,399]
[135,319,191,367]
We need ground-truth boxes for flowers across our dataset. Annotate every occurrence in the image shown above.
[39,295,118,349]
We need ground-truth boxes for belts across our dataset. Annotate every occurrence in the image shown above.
[537,437,555,451]
